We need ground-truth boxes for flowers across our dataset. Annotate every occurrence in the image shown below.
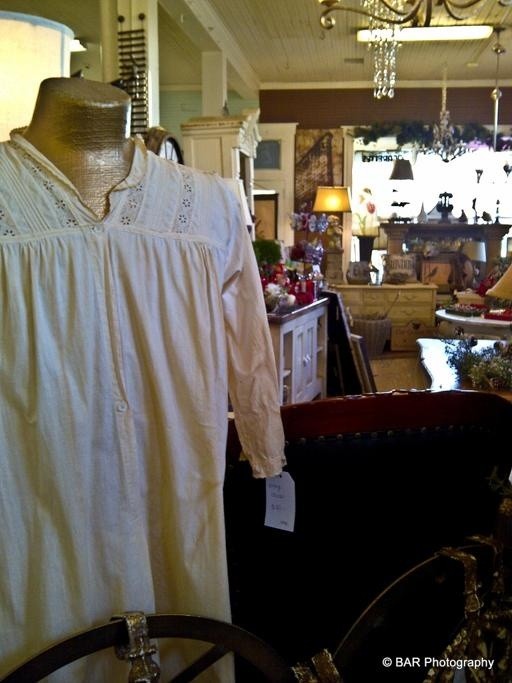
[441,329,512,387]
[289,205,328,266]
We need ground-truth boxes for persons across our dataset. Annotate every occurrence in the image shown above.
[0,74,291,682]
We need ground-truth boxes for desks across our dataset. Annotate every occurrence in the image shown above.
[416,338,512,403]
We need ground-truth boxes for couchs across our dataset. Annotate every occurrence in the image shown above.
[224,391,512,683]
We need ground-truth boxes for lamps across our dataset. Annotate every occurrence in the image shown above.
[319,0,503,163]
[312,186,352,214]
[485,263,512,298]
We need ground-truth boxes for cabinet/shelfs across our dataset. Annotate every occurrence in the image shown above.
[332,284,439,351]
[253,122,299,250]
[267,298,331,406]
